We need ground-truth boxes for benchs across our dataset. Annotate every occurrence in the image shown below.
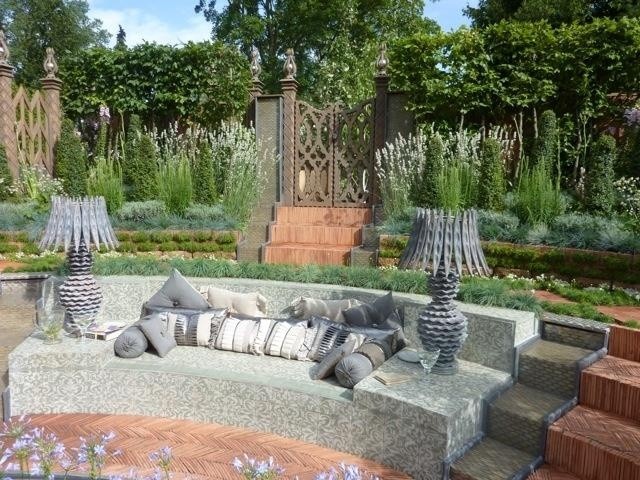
[4,273,540,480]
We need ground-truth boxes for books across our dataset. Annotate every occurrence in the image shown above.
[373,372,411,385]
[85,328,123,341]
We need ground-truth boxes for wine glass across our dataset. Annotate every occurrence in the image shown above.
[36,305,67,345]
[415,345,441,386]
[73,312,93,345]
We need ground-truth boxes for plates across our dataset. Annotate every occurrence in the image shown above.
[397,351,420,363]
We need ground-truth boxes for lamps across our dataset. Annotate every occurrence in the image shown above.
[396,207,494,377]
[37,195,121,341]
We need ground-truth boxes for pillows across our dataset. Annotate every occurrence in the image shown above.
[113,266,412,388]
